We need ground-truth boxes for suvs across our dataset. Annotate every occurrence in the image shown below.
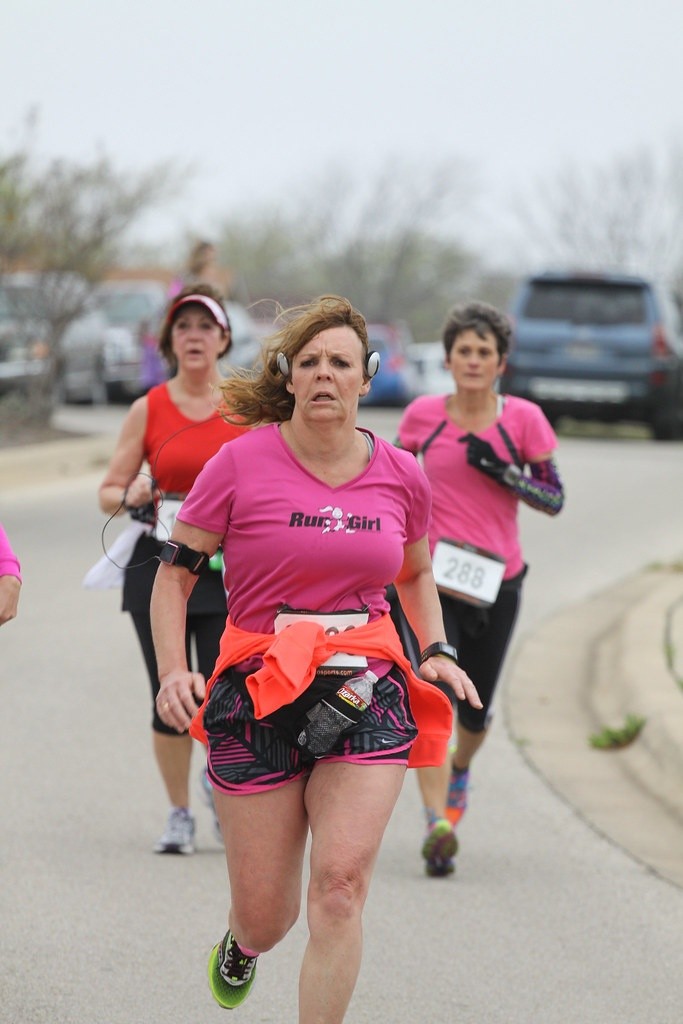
[491,265,683,444]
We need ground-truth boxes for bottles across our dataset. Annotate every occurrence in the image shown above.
[296,670,379,759]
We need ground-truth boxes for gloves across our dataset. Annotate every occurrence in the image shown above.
[458,431,508,480]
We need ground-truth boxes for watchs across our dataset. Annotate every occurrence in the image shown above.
[420,641,459,665]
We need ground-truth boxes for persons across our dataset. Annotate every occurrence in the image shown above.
[0,524,22,625]
[150,294,484,1023]
[101,284,256,857]
[391,304,565,875]
[169,244,216,298]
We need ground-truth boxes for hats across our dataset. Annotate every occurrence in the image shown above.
[165,294,229,332]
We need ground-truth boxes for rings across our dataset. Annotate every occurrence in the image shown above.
[162,702,169,711]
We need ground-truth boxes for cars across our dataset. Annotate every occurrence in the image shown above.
[0,269,281,407]
[358,321,411,411]
[400,342,500,409]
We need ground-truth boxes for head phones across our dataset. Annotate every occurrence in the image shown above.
[276,351,380,381]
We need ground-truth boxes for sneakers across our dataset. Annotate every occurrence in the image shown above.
[156,809,195,854]
[446,750,469,821]
[208,929,259,1008]
[202,773,224,842]
[423,819,459,876]
